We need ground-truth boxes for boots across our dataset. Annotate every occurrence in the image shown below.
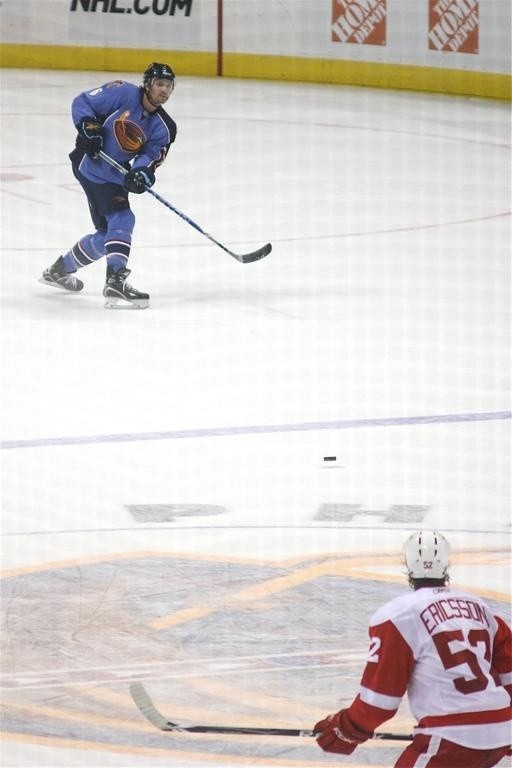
[104,266,149,299]
[42,255,83,291]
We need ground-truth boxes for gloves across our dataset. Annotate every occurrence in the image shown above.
[125,166,155,194]
[76,116,103,158]
[312,708,373,756]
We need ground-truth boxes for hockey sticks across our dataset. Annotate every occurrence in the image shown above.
[130,682,414,740]
[98,151,272,263]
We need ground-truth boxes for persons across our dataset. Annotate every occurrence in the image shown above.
[311,530,511,768]
[41,60,177,303]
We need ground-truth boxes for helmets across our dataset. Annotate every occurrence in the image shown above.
[402,530,452,582]
[143,63,175,105]
